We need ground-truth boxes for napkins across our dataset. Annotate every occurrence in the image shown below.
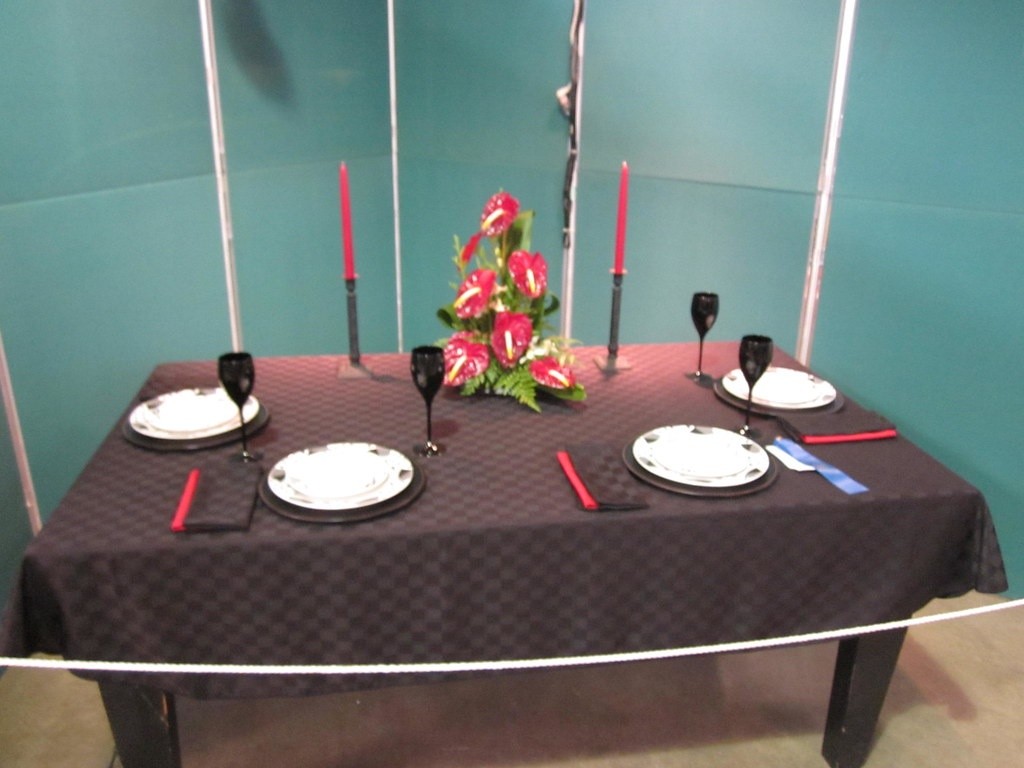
[172,464,261,532]
[776,411,897,445]
[557,441,650,511]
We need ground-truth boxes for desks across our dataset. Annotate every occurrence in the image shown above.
[0,343,1010,768]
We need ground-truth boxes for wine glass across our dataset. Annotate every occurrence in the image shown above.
[411,345,446,456]
[734,335,775,438]
[685,292,719,386]
[217,352,264,465]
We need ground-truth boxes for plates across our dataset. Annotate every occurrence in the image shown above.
[724,366,837,412]
[266,442,414,512]
[121,402,269,452]
[128,389,260,440]
[259,461,426,525]
[622,439,779,498]
[712,378,846,417]
[633,423,770,488]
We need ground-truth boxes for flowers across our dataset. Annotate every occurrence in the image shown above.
[436,191,587,412]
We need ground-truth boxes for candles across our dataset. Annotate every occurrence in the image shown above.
[614,160,628,273]
[339,161,356,280]
